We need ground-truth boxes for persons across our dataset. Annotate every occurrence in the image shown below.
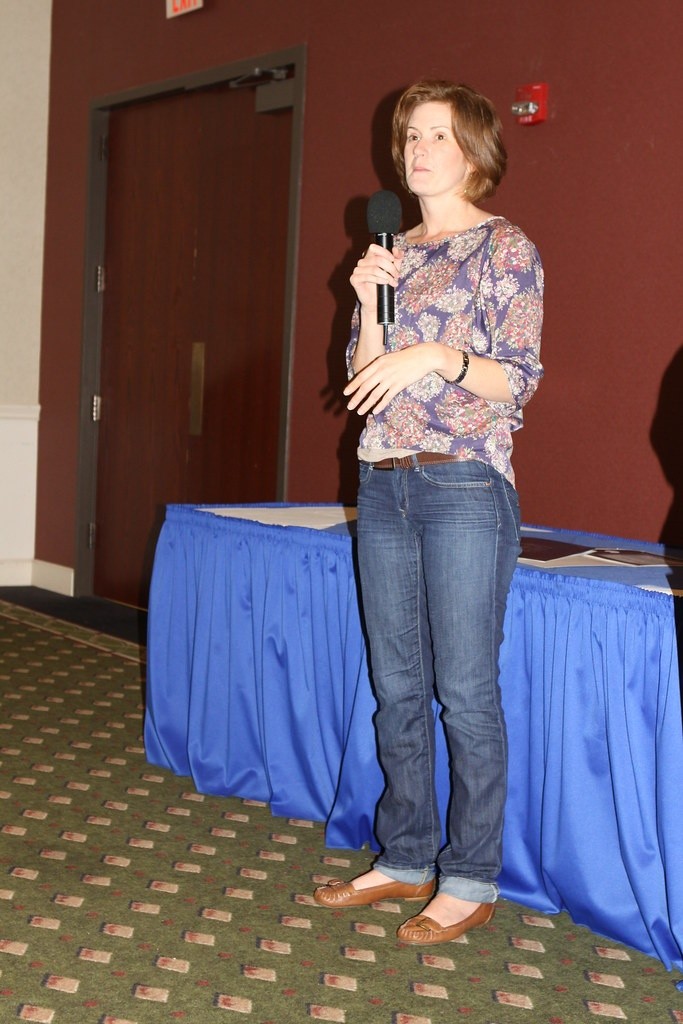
[313,82,542,945]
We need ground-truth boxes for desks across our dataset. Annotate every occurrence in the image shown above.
[144,495,683,983]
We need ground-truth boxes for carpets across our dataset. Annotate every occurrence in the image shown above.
[1,598,680,1024]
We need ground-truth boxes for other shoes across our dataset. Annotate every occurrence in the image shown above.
[396,891,496,946]
[313,868,438,908]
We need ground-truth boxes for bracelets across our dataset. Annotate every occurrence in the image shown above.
[442,349,469,385]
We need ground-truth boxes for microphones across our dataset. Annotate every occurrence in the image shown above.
[367,191,402,346]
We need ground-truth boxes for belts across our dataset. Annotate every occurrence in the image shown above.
[373,451,468,468]
